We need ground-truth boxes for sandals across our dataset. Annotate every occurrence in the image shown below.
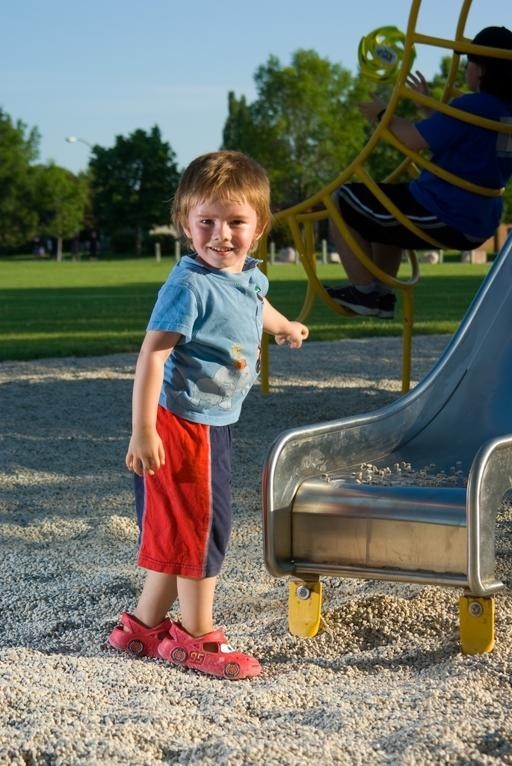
[157,622,261,679]
[108,611,172,659]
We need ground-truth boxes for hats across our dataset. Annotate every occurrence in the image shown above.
[454,27,512,67]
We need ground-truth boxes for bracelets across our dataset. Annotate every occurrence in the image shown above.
[376,107,385,120]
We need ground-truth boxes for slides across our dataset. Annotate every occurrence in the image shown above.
[262,227,511,596]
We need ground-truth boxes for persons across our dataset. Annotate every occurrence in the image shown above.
[108,150,310,678]
[30,221,106,263]
[321,26,512,320]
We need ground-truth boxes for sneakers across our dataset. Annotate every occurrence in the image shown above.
[322,284,397,319]
